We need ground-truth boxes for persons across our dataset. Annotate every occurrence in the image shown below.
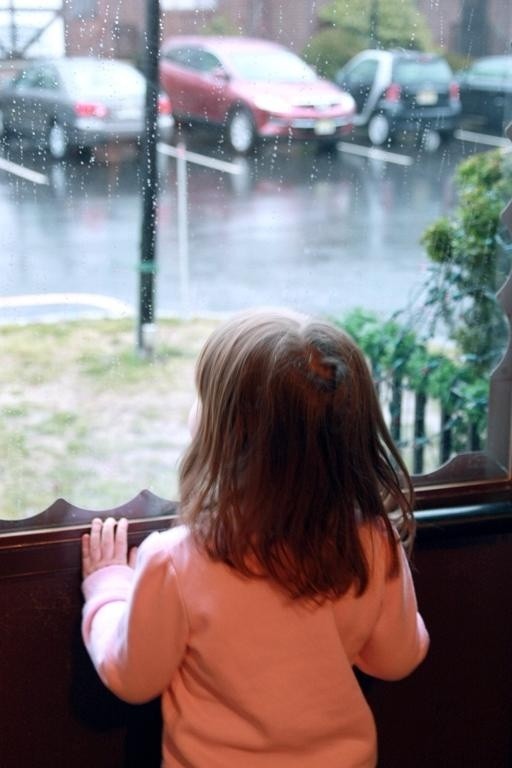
[81,309,432,768]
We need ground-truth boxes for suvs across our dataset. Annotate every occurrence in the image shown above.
[155,27,358,158]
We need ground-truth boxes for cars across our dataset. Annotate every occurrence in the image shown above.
[330,41,468,152]
[0,54,177,163]
[453,50,512,128]
